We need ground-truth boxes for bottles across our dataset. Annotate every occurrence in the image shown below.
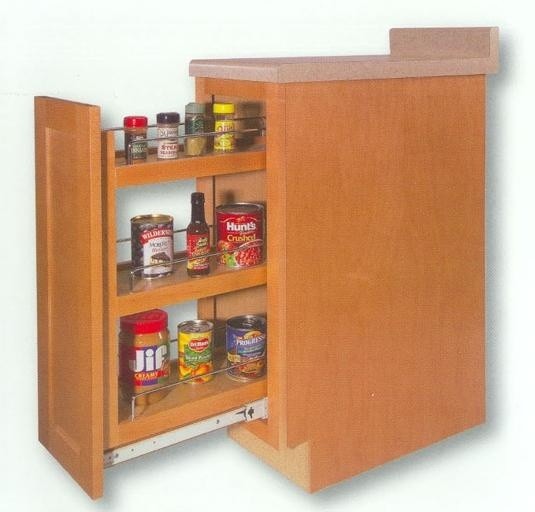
[186,192,212,277]
[123,101,236,166]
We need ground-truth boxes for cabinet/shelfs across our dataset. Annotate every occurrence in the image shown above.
[35,27,500,499]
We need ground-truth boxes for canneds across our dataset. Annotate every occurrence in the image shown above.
[177,319,215,385]
[215,202,264,268]
[225,314,266,383]
[130,213,175,281]
[119,309,171,405]
[211,102,236,153]
